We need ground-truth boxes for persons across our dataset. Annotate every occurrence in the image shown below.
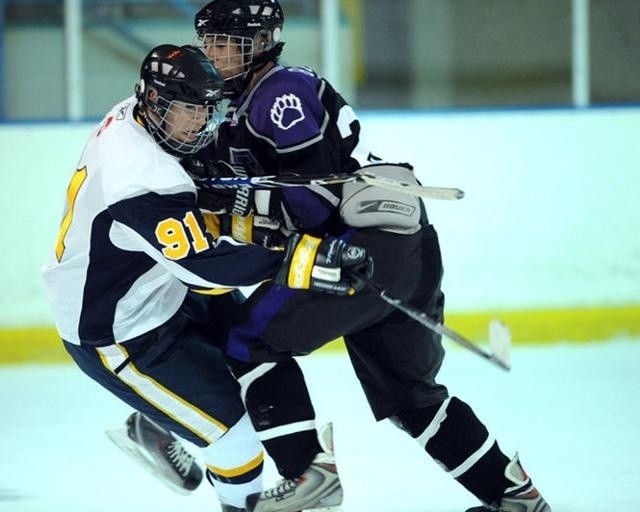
[181,0,559,511]
[44,43,384,512]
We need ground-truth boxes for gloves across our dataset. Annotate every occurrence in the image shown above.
[250,214,290,252]
[282,231,376,295]
[176,155,255,218]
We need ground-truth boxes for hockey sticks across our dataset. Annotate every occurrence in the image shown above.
[192,172,465,200]
[351,271,512,371]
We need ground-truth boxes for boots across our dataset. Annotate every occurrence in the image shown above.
[245,421,344,512]
[464,450,553,512]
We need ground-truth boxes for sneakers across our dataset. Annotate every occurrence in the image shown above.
[124,411,203,492]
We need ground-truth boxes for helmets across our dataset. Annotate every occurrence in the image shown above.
[134,43,225,154]
[193,0,285,101]
